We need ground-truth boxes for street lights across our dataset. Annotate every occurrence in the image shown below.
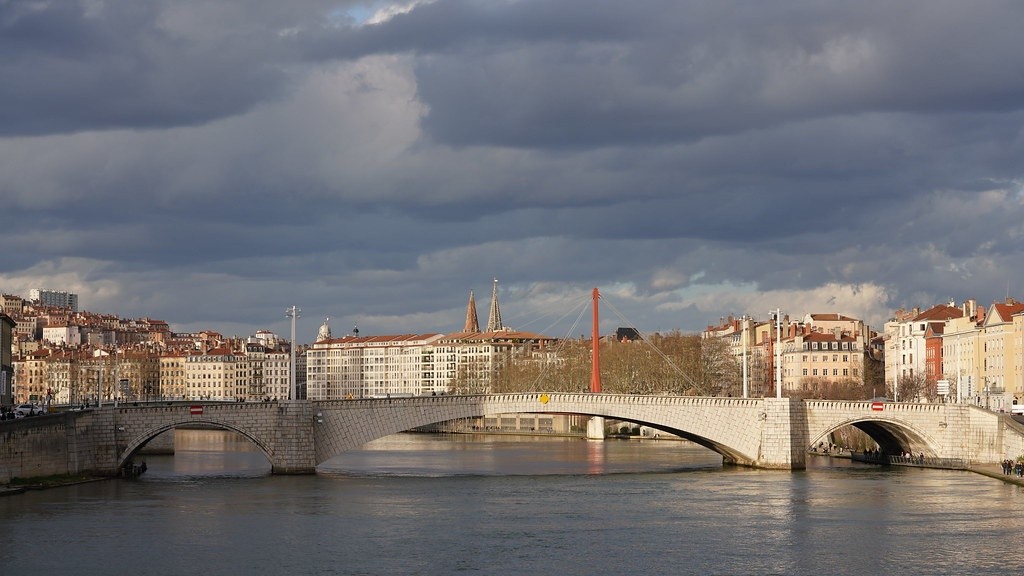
[96,336,104,406]
[284,304,303,400]
[733,313,754,399]
[767,308,785,398]
[112,342,119,407]
[889,342,900,402]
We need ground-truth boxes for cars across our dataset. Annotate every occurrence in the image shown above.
[13,404,48,416]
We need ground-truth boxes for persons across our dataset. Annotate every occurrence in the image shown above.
[812,441,924,464]
[0,410,36,421]
[431,390,436,401]
[234,395,278,409]
[1001,459,1024,478]
